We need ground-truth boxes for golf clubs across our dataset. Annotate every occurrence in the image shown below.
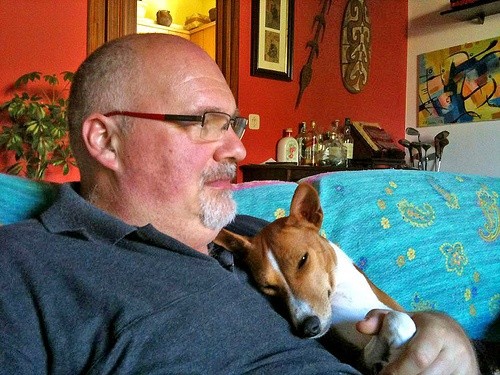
[398,126,450,172]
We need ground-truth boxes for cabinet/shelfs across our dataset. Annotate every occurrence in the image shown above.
[137,21,216,61]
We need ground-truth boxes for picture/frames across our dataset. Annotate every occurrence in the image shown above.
[250,0,294,81]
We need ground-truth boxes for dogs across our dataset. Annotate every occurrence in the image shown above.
[214,182,417,375]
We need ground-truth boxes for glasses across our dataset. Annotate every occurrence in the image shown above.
[103,111,249,142]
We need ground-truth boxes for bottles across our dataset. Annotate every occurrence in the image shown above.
[330,132,342,165]
[333,120,341,140]
[277,128,298,165]
[305,120,318,164]
[343,118,354,166]
[324,131,333,164]
[296,121,305,164]
[317,134,326,165]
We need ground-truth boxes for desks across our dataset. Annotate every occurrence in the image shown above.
[240,163,361,181]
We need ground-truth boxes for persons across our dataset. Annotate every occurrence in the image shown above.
[0,32,482,375]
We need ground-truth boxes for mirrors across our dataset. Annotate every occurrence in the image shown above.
[107,0,240,111]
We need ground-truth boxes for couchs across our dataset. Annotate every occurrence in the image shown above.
[0,169,500,342]
